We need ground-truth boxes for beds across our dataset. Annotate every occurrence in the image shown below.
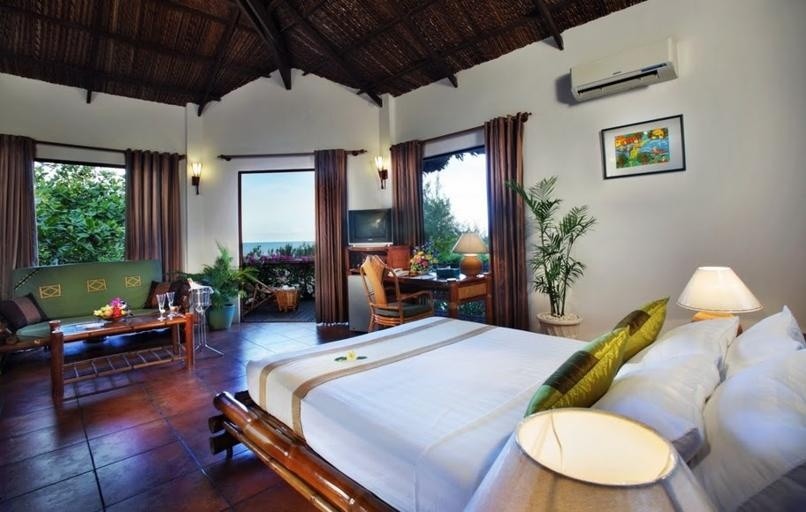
[208,316,806,512]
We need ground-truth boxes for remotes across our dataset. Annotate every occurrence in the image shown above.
[84,322,104,329]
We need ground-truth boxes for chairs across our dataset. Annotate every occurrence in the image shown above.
[358,255,433,331]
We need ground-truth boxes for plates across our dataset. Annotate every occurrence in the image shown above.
[100,315,127,321]
[101,322,128,328]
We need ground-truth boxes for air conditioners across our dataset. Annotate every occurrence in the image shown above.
[568,36,678,103]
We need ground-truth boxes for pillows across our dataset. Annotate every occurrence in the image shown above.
[598,317,739,458]
[524,299,668,421]
[143,278,185,308]
[694,305,806,512]
[0,291,48,330]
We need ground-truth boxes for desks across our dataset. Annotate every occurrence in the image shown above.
[388,266,494,322]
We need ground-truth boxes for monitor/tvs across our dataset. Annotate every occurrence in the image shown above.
[347,208,394,247]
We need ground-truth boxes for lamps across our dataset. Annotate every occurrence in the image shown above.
[679,265,764,327]
[193,162,203,190]
[462,404,715,512]
[372,154,387,188]
[452,231,487,275]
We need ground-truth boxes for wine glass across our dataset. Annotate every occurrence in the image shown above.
[166,291,176,318]
[155,292,166,320]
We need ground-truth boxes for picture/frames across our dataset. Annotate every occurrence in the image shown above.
[601,115,686,179]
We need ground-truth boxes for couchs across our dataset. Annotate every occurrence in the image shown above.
[0,258,210,370]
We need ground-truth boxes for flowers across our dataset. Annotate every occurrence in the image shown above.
[93,298,130,322]
[410,252,439,273]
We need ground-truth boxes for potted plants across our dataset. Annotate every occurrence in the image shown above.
[179,243,256,330]
[504,176,597,338]
